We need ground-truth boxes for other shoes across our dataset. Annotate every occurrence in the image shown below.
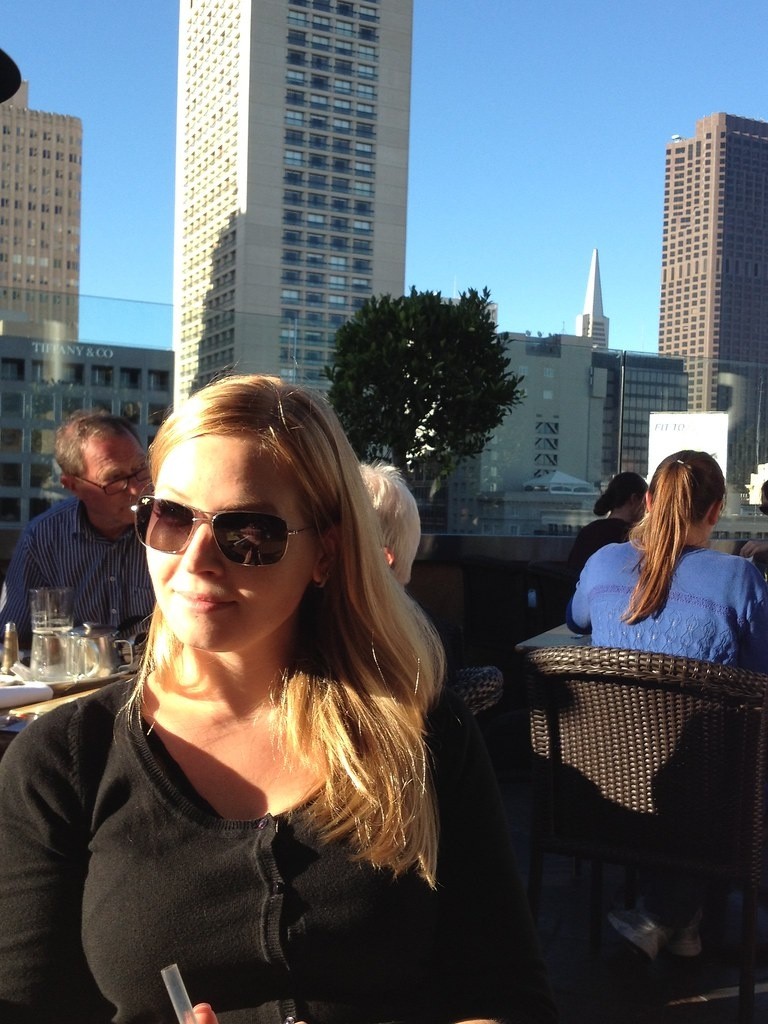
[607,906,703,957]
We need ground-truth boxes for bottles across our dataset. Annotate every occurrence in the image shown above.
[1,621,20,676]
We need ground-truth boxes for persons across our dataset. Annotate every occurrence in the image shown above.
[0,407,157,652]
[0,354,552,1024]
[565,451,767,961]
[354,458,422,593]
[738,481,768,558]
[565,470,648,573]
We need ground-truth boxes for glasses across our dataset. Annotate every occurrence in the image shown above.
[72,467,152,496]
[135,495,311,566]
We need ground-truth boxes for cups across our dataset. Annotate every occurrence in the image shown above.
[28,586,76,634]
[69,621,135,678]
[29,634,101,682]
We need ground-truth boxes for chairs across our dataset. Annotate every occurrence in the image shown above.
[518,646,768,1024]
